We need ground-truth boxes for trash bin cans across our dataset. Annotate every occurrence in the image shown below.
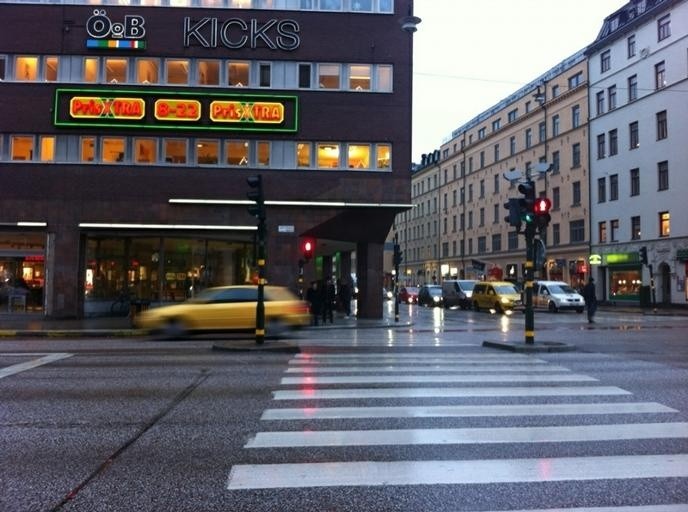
[129,300,150,329]
[639,285,651,308]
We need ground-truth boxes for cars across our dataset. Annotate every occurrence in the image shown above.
[522,280,586,313]
[418,284,443,307]
[399,287,420,304]
[139,285,312,340]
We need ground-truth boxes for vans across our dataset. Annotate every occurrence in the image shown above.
[472,281,522,313]
[442,280,479,310]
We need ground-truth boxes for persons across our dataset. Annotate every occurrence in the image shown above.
[306,281,322,326]
[583,276,600,324]
[337,278,353,320]
[575,278,584,296]
[321,275,336,324]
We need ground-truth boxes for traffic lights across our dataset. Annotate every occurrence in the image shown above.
[639,246,647,264]
[394,244,403,265]
[246,174,263,216]
[503,181,551,226]
